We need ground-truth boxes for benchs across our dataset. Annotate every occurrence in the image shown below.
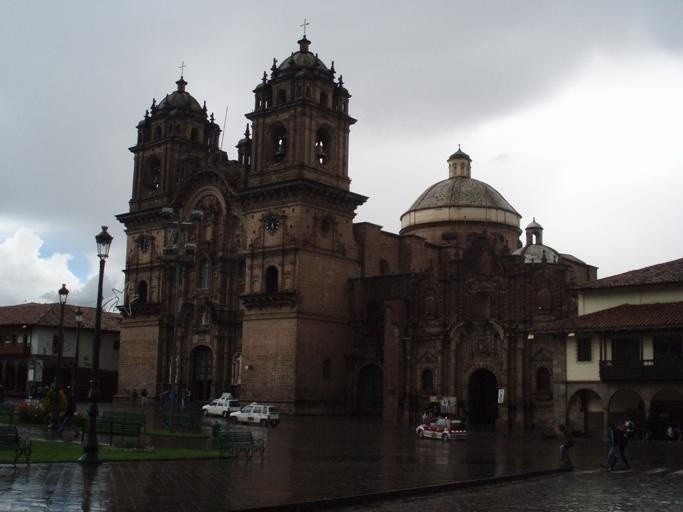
[0,403,15,426]
[78,417,142,454]
[0,425,31,467]
[212,431,264,464]
[101,411,146,447]
[160,410,201,435]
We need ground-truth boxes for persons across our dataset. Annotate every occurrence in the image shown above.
[556,425,572,463]
[602,423,628,472]
[611,425,631,471]
[131,387,191,411]
[56,393,79,438]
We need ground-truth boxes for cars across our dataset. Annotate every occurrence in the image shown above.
[200,393,241,418]
[228,401,280,428]
[415,416,466,443]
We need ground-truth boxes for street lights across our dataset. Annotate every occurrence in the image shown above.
[47,284,70,430]
[72,306,84,397]
[77,225,114,464]
[157,200,206,422]
